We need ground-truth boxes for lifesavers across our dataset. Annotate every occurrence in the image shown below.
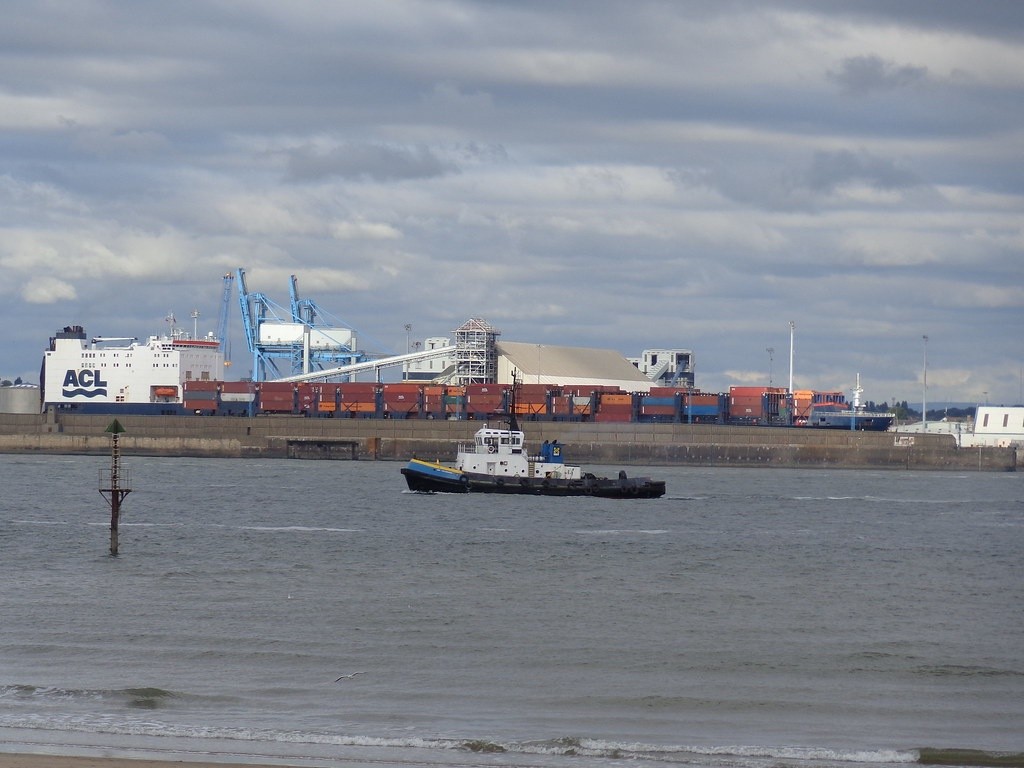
[487,446,495,453]
[567,482,577,491]
[464,481,473,490]
[621,485,628,494]
[503,460,508,466]
[520,477,531,489]
[540,479,550,489]
[630,484,640,495]
[496,478,505,487]
[584,485,600,495]
[458,474,469,484]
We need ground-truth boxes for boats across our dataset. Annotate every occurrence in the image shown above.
[808,373,895,431]
[400,367,666,499]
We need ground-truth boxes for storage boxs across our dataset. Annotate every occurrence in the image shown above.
[183,376,843,426]
